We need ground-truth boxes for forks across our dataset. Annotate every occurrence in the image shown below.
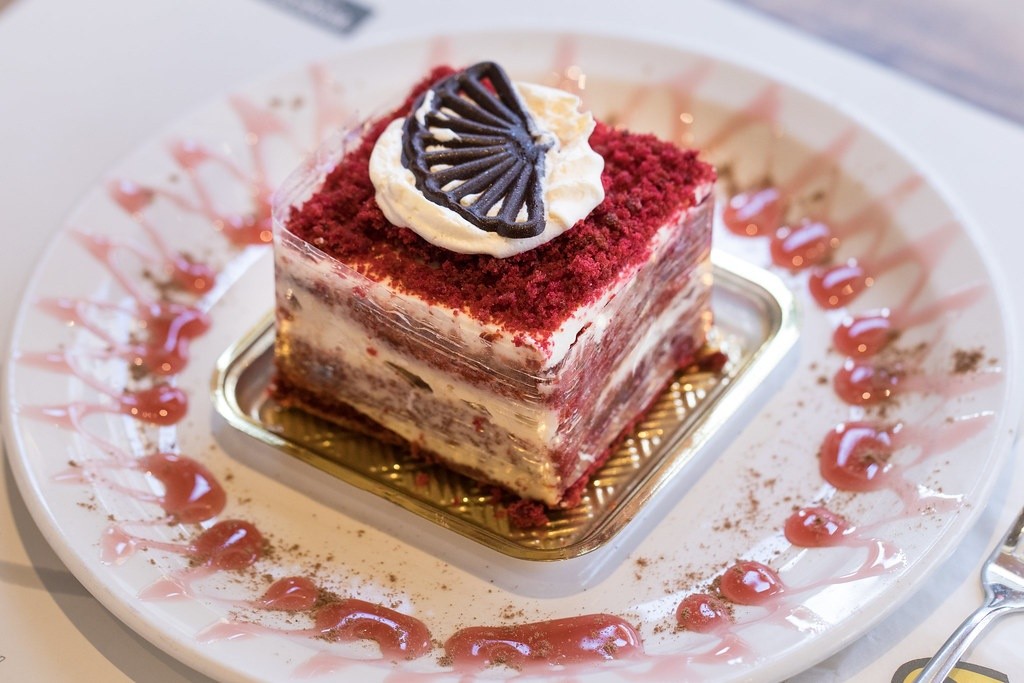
[911,505,1024,683]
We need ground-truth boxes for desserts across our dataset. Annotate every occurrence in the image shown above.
[268,63,719,510]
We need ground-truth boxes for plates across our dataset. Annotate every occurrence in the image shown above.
[0,24,1012,683]
